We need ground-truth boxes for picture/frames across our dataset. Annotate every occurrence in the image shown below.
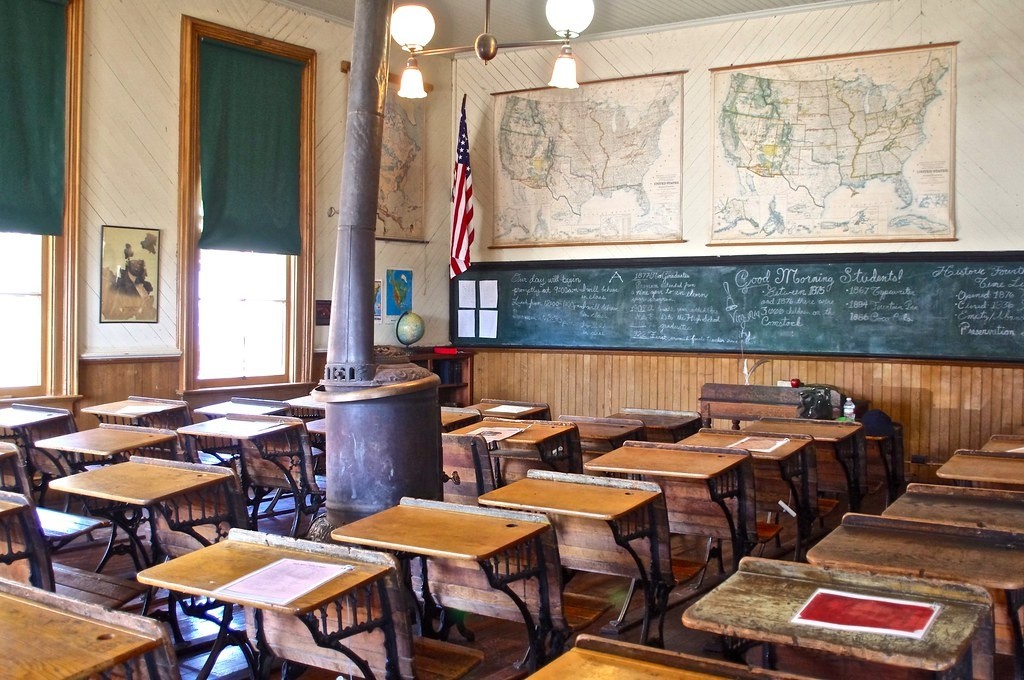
[99,225,160,323]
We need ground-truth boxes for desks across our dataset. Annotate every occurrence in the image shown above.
[0,381,1024,680]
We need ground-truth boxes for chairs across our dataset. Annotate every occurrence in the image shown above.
[0,397,1024,680]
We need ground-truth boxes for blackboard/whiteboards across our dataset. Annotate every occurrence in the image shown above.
[446,249,1024,363]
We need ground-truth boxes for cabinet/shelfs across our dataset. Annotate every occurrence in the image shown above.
[409,353,474,408]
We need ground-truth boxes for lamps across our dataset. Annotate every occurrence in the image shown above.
[389,0,594,99]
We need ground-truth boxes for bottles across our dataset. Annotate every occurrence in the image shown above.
[843,397,856,422]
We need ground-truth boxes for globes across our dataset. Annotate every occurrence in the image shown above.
[395,312,425,347]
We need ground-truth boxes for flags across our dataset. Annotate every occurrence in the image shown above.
[449,93,474,279]
